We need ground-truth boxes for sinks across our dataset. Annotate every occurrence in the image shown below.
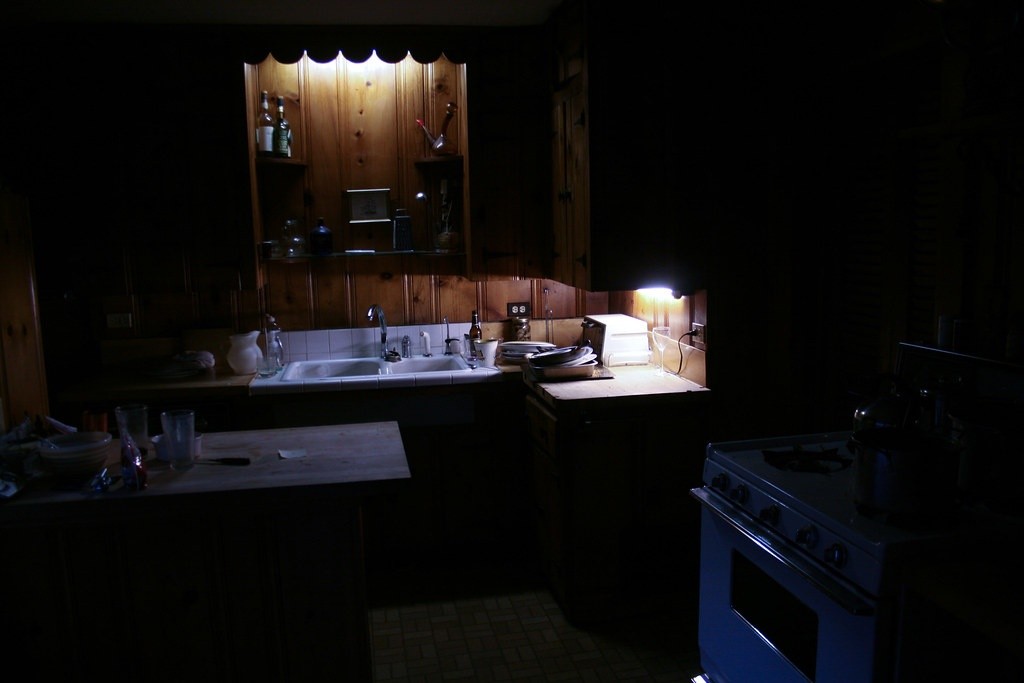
[386,353,472,379]
[281,356,385,380]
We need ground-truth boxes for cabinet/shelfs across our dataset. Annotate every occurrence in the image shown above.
[30,12,792,623]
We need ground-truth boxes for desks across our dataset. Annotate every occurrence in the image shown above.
[0,419,412,681]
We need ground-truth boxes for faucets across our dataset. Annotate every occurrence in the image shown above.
[365,304,388,358]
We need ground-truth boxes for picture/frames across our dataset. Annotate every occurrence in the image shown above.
[344,187,391,225]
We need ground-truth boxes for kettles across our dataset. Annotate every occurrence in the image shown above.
[851,373,921,450]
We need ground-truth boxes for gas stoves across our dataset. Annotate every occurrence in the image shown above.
[702,332,1023,599]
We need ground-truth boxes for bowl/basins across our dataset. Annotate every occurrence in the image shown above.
[151,430,204,462]
[34,430,112,480]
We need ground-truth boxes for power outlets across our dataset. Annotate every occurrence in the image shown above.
[507,302,531,318]
[691,321,704,344]
[108,313,131,329]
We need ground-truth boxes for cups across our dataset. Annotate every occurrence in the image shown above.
[472,338,498,368]
[159,409,197,468]
[115,405,149,460]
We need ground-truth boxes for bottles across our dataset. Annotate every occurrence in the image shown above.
[225,330,263,375]
[265,316,284,373]
[468,310,483,360]
[310,217,334,256]
[256,90,293,159]
[391,208,411,251]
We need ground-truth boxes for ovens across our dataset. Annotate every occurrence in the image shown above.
[687,486,904,683]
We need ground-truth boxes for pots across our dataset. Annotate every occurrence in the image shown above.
[845,425,969,514]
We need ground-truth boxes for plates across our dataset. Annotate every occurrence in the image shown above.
[498,340,598,367]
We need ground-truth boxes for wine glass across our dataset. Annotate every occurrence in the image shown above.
[653,326,670,376]
